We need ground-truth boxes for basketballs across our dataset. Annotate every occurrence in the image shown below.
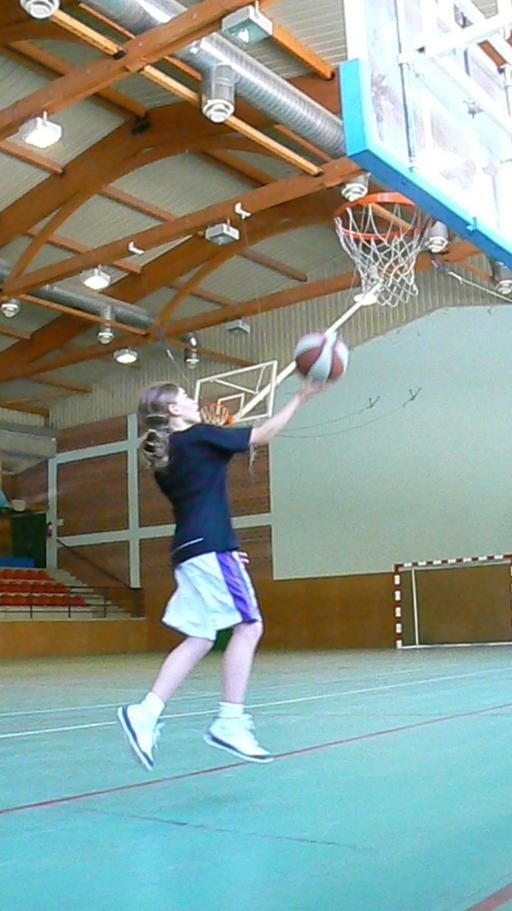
[297,332,348,379]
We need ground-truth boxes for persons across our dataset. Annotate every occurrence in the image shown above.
[114,376,328,771]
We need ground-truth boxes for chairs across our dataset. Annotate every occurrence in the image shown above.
[0,569,85,607]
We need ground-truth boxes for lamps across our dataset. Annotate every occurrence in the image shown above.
[113,349,138,364]
[205,223,240,246]
[225,319,251,337]
[81,264,112,291]
[19,117,63,150]
[221,5,273,47]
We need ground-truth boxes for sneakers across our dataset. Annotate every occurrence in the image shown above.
[202,715,273,762]
[118,705,157,771]
